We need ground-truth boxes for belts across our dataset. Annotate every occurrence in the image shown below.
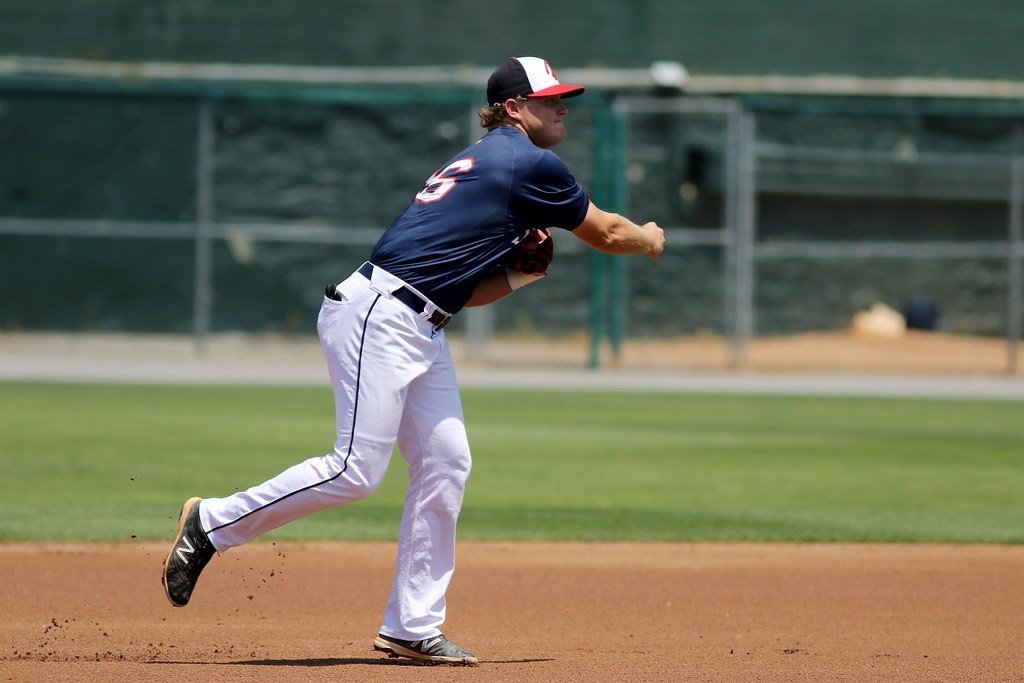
[359,262,452,332]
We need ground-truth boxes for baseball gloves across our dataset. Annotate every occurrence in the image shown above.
[482,228,554,277]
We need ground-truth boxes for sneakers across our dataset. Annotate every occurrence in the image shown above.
[374,634,478,664]
[162,497,217,607]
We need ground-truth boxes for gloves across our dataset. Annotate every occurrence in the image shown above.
[499,227,553,291]
[325,284,341,301]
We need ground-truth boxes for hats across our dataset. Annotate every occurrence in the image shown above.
[487,57,585,108]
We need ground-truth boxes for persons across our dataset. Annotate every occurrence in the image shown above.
[161,54,665,666]
